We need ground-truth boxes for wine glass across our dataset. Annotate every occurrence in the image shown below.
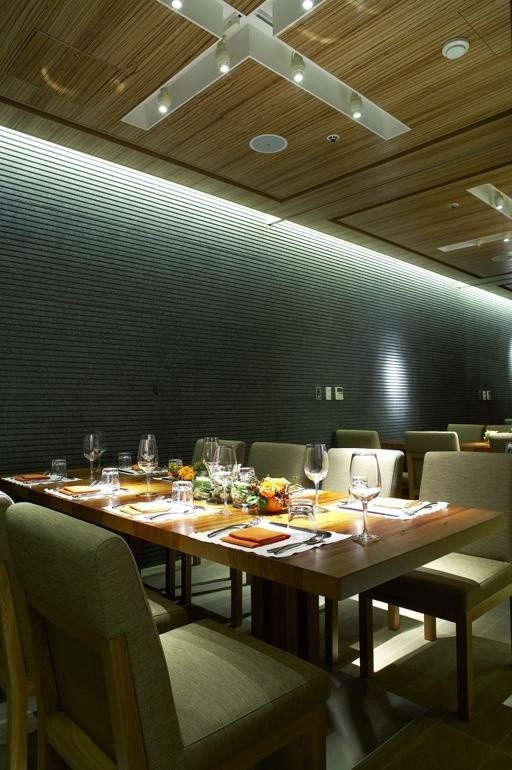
[348,452,381,546]
[82,430,335,516]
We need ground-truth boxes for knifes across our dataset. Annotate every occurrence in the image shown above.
[270,521,331,538]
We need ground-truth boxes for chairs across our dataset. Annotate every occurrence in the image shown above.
[0,424,512,770]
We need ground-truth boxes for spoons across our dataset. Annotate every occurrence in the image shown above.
[272,532,324,554]
[207,518,261,539]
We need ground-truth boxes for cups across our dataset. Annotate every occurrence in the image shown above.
[287,498,317,539]
[51,459,68,484]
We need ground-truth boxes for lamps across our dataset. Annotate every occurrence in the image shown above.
[435,180,511,250]
[120,0,410,143]
